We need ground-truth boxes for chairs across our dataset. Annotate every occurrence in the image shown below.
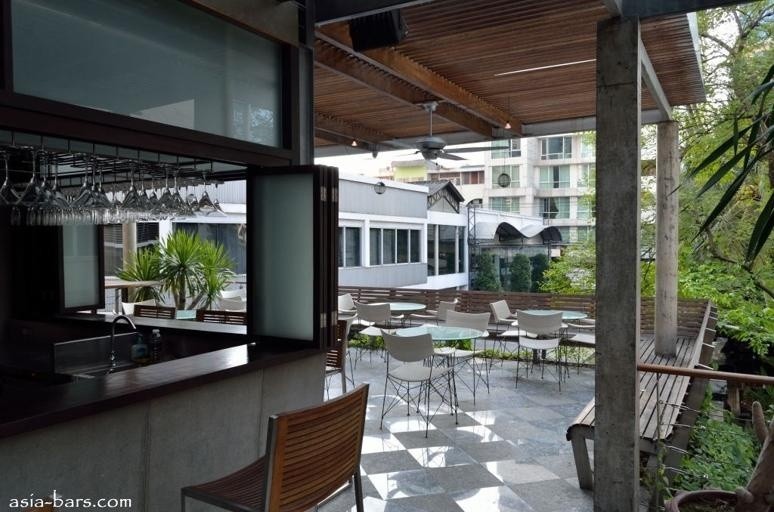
[219,299,248,310]
[180,382,369,511]
[122,299,157,316]
[221,289,247,304]
[133,305,174,318]
[196,309,247,325]
[325,320,348,394]
[339,293,596,438]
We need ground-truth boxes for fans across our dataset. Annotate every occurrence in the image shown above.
[391,101,509,160]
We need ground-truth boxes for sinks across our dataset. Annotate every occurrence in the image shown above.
[74,364,141,377]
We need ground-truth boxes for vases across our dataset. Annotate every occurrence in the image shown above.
[664,490,742,512]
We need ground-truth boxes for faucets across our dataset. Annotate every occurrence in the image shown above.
[107,315,137,366]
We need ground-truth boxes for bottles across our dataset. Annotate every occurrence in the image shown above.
[130,333,148,361]
[151,329,163,361]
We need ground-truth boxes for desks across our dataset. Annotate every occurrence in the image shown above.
[105,281,160,313]
[176,309,197,320]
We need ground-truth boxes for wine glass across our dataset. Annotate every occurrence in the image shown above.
[0,142,230,227]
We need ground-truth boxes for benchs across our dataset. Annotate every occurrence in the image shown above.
[338,286,718,491]
